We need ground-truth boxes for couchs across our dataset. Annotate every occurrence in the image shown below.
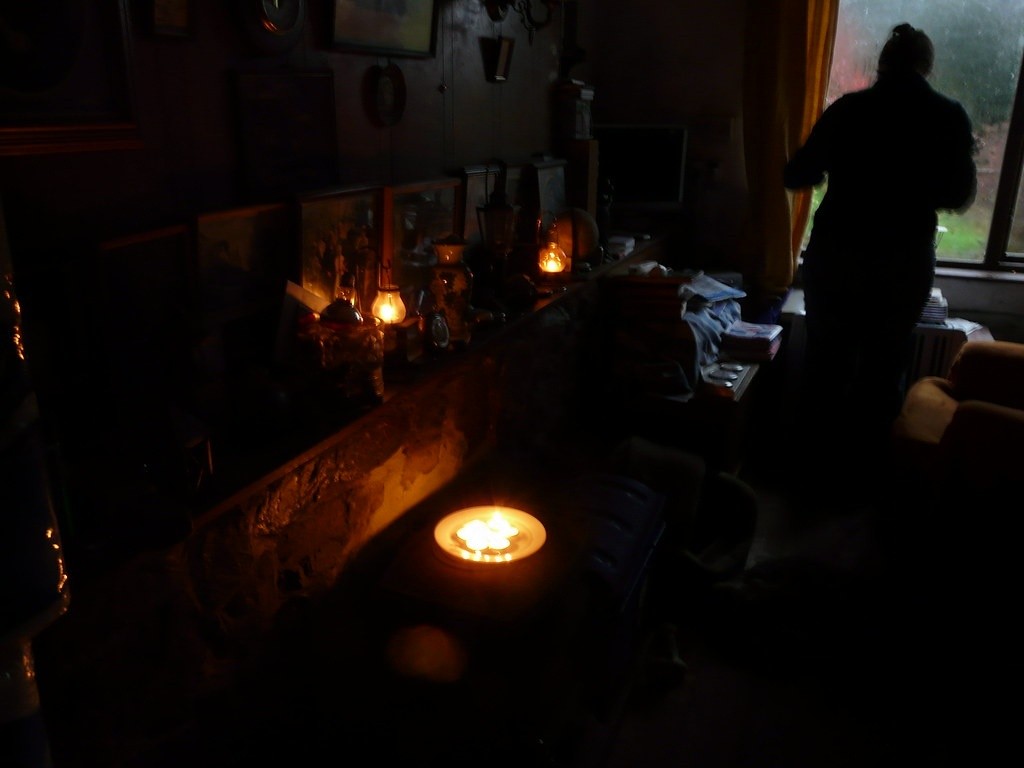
[894,341,1024,509]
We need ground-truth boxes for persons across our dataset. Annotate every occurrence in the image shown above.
[778,23,976,527]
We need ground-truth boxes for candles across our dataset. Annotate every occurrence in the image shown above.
[454,522,518,554]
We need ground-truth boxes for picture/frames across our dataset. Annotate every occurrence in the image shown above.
[329,0,442,59]
[491,35,516,81]
[148,0,198,41]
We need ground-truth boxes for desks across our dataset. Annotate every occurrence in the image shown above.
[779,291,967,421]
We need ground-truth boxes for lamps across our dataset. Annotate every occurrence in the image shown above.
[372,255,407,328]
[536,219,568,273]
